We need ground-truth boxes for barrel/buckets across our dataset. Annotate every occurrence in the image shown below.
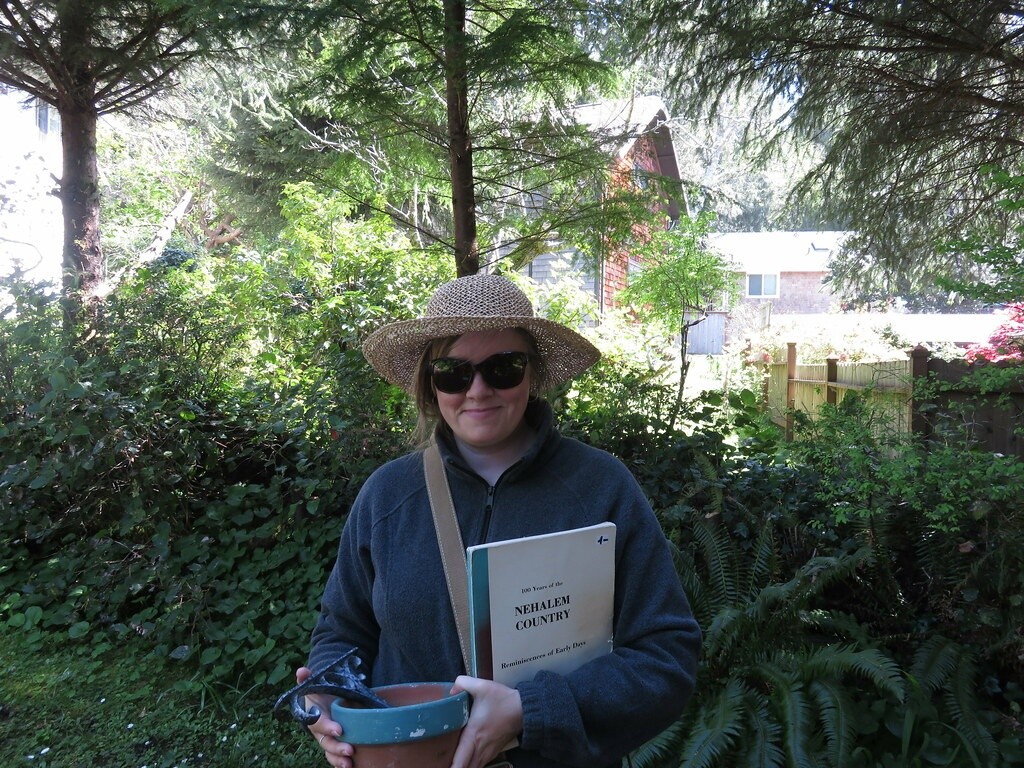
[331,681,469,768]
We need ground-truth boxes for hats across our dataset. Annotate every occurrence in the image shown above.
[362,274,602,398]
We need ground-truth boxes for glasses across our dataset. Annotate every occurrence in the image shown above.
[427,352,534,393]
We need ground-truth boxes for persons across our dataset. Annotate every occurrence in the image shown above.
[296,274,702,768]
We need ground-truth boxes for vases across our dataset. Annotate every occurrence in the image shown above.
[330,682,470,768]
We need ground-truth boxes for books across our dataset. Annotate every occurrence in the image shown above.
[466,522,616,753]
